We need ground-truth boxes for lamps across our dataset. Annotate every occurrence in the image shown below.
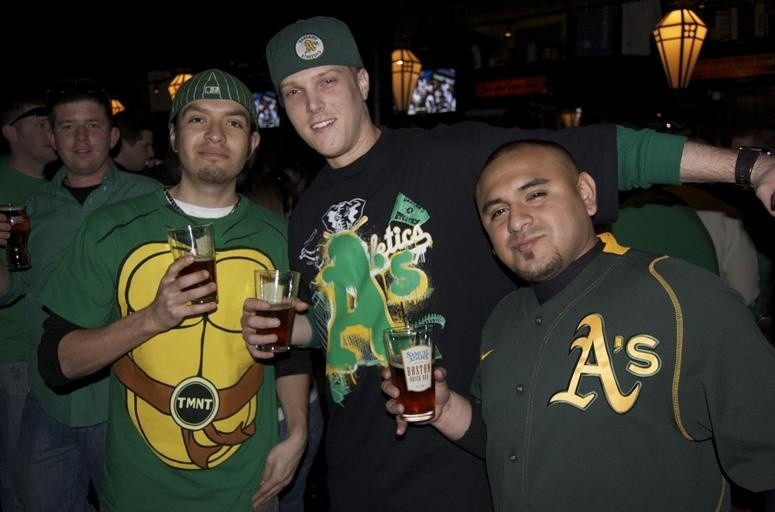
[650,3,710,93]
[165,70,194,106]
[110,98,126,119]
[390,49,424,114]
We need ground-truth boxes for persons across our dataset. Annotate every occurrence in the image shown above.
[38,71,310,509]
[1,82,164,510]
[242,16,775,511]
[1,91,61,508]
[111,108,154,169]
[384,141,775,511]
[614,184,771,317]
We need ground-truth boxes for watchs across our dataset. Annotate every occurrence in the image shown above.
[736,147,771,191]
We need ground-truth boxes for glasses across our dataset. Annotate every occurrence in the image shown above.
[9,103,53,126]
[46,79,109,101]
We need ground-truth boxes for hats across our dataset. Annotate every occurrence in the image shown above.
[265,16,364,108]
[168,68,259,132]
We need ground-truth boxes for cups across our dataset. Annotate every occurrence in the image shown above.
[0,204,33,269]
[164,224,220,304]
[382,326,440,424]
[253,269,302,348]
